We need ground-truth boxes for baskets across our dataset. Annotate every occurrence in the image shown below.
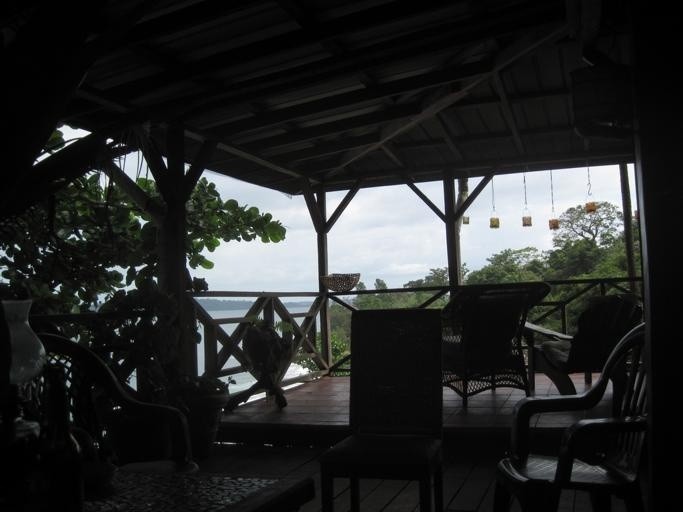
[320,273,360,292]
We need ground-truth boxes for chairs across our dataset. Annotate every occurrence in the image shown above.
[440,282,551,407]
[534,292,643,395]
[495,323,647,512]
[2,333,198,507]
[317,308,441,507]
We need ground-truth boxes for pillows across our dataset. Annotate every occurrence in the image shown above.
[543,338,577,378]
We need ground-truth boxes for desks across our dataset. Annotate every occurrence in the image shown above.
[76,469,316,512]
